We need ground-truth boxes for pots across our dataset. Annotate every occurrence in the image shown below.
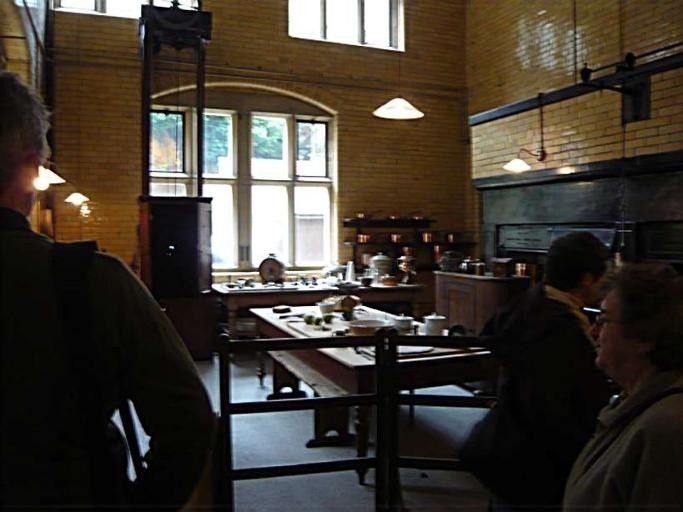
[368,251,393,275]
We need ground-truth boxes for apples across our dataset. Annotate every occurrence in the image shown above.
[304,315,314,324]
[315,317,322,325]
[324,314,333,323]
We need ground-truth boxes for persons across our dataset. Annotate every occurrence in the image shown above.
[0,74,214,512]
[479,231,615,444]
[562,263,683,512]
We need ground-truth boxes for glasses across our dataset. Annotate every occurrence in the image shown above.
[595,316,624,328]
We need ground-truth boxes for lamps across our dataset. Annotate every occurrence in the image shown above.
[371,2,425,124]
[65,6,91,209]
[502,146,547,174]
[33,160,66,193]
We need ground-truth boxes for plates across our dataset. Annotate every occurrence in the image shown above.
[356,344,434,359]
[333,281,361,290]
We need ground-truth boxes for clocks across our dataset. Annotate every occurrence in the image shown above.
[258,252,285,284]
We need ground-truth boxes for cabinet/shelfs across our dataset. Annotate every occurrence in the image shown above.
[136,194,214,294]
[433,266,534,340]
[153,291,215,361]
[344,209,443,272]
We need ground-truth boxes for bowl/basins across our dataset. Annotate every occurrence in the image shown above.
[350,318,391,337]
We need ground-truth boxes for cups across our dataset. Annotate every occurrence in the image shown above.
[395,314,414,331]
[380,273,398,286]
[344,260,356,281]
[472,262,485,275]
[365,268,379,284]
[422,312,447,336]
[318,301,335,314]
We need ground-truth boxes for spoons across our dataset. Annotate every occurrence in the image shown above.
[279,313,305,318]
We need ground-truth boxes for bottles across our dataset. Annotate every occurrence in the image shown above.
[460,256,480,275]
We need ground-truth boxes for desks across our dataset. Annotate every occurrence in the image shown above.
[213,275,426,339]
[246,297,496,489]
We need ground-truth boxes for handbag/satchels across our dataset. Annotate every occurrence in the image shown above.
[465,403,533,502]
